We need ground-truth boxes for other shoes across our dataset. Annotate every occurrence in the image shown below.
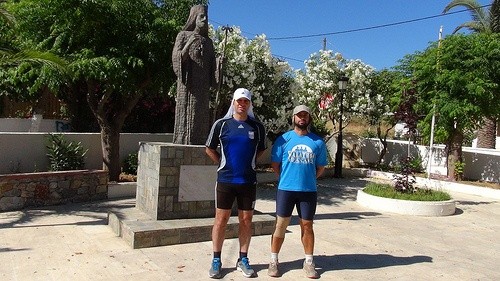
[268,262,281,277]
[303,261,320,278]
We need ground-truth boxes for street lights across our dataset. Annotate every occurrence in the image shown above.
[334,76,350,178]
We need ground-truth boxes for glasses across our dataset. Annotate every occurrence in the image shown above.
[295,113,308,118]
[237,100,247,103]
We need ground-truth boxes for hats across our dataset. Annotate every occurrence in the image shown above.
[293,105,311,115]
[234,88,251,101]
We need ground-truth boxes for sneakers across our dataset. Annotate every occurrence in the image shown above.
[236,257,257,278]
[209,258,222,278]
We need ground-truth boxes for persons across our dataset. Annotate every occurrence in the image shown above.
[204,88,269,278]
[170,4,217,145]
[268,105,329,278]
